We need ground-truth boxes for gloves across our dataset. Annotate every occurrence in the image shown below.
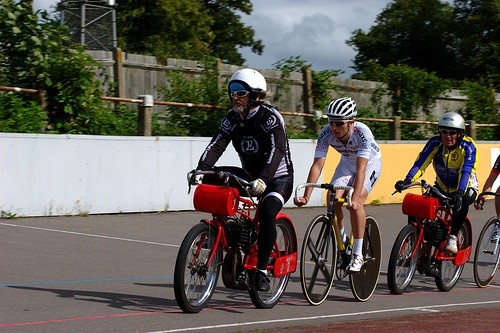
[450,194,461,212]
[244,179,266,197]
[186,170,205,185]
[395,180,409,193]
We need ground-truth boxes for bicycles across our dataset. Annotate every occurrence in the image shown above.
[474,191,500,289]
[172,170,298,315]
[386,178,474,296]
[294,183,383,307]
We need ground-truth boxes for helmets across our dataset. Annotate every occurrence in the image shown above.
[438,112,465,133]
[227,68,267,99]
[327,97,357,120]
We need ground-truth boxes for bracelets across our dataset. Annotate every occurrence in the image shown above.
[303,198,307,203]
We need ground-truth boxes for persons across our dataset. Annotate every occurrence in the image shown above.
[474,154,500,243]
[294,97,382,272]
[187,69,293,291]
[394,112,479,267]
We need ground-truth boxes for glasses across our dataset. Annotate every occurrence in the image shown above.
[230,90,254,98]
[328,120,354,126]
[438,127,461,135]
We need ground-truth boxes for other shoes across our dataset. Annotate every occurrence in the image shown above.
[491,228,499,243]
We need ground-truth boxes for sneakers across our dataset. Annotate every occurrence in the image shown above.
[397,254,412,267]
[195,239,210,249]
[445,235,458,254]
[346,255,364,272]
[342,233,349,245]
[256,270,270,292]
